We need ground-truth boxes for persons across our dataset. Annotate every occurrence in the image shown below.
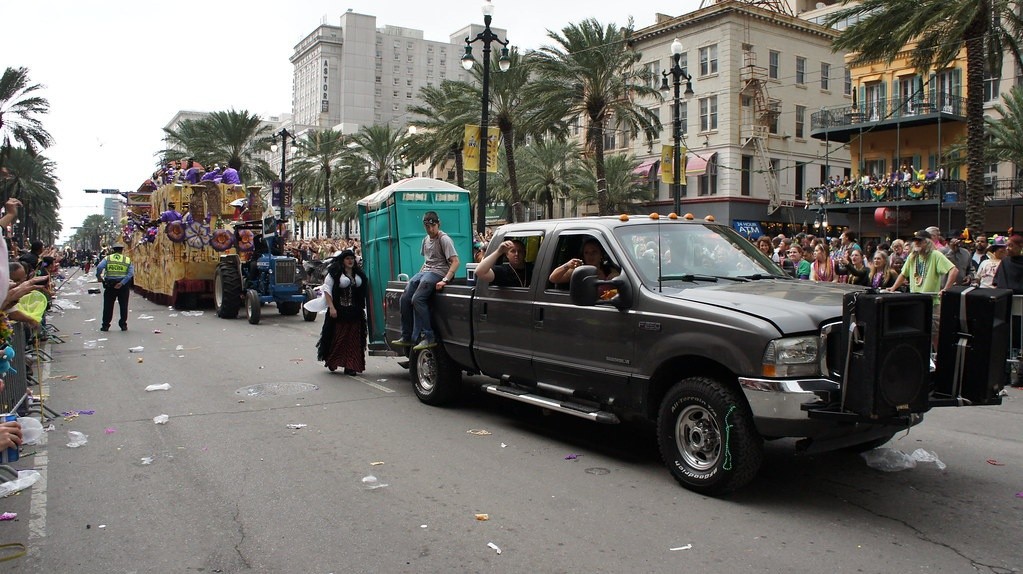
[62,244,107,270]
[126,202,190,241]
[96,241,134,331]
[475,240,533,288]
[0,197,60,452]
[549,237,619,299]
[150,157,239,191]
[391,212,458,348]
[473,230,494,262]
[284,236,363,290]
[636,226,1023,356]
[820,165,944,203]
[306,247,367,376]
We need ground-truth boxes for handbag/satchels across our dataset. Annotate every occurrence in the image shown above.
[327,300,358,321]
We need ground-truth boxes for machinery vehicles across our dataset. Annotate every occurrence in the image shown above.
[118,183,317,325]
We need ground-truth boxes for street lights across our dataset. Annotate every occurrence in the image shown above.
[407,122,417,178]
[461,0,510,236]
[99,217,116,244]
[657,36,694,216]
[814,193,828,238]
[270,127,298,234]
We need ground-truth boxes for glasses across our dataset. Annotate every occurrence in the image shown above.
[423,218,435,225]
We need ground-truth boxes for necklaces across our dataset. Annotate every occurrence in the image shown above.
[813,257,834,281]
[510,263,526,287]
[914,254,925,286]
[348,274,353,278]
[847,265,864,284]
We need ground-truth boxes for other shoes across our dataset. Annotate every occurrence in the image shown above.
[343,368,356,376]
[328,365,337,372]
[391,337,412,346]
[100,327,108,331]
[413,338,437,350]
[120,326,128,330]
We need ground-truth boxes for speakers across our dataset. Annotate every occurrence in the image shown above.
[840,290,934,420]
[930,284,1013,403]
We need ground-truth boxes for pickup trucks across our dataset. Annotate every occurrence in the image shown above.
[383,213,937,496]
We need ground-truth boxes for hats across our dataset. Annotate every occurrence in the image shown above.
[912,230,931,240]
[113,243,124,248]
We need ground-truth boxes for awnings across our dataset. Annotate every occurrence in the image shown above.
[630,158,660,183]
[657,151,717,180]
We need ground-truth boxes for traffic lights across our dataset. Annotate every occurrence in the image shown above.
[294,223,299,235]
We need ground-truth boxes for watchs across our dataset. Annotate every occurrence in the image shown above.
[442,278,449,283]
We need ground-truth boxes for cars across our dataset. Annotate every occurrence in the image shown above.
[85,255,98,266]
